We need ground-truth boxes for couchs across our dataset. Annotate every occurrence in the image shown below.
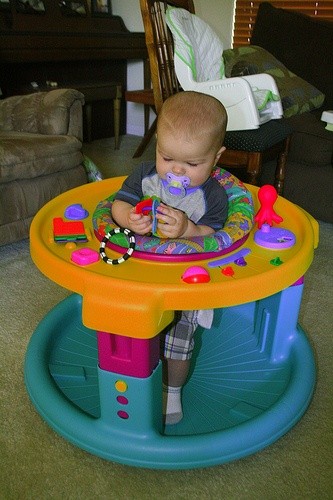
[0,89,87,248]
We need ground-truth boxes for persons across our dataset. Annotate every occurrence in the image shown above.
[110,90,229,425]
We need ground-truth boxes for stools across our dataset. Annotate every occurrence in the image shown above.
[47,78,122,150]
[126,88,184,158]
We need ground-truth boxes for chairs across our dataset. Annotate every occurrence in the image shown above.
[133,0,290,195]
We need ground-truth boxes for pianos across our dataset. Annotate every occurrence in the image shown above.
[0,9,153,144]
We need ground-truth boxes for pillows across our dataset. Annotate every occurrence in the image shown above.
[163,5,324,130]
